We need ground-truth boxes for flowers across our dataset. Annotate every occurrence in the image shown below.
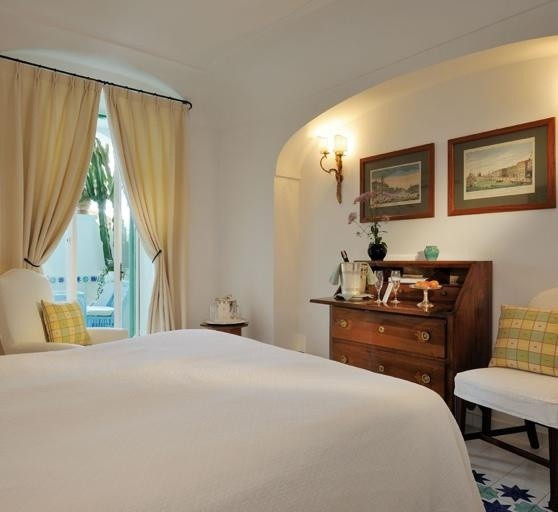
[347,191,396,243]
[97,258,114,299]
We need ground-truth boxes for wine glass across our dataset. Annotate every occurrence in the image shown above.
[390,271,401,304]
[373,270,384,303]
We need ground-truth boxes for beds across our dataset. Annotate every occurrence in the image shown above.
[0,328,485,511]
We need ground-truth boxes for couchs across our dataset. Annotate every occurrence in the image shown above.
[0,268,129,354]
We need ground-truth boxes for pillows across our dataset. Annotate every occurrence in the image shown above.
[41,299,93,346]
[489,303,558,376]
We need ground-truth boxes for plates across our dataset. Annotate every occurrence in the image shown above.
[204,320,249,325]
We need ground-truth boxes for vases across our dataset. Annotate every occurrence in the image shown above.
[108,271,115,281]
[368,242,388,261]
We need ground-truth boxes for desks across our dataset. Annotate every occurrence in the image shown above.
[199,321,249,336]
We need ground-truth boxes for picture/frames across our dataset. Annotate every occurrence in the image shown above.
[360,142,435,223]
[447,117,557,216]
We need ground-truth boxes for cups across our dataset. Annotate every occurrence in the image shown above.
[208,294,240,322]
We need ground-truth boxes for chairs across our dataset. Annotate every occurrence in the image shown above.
[453,287,557,511]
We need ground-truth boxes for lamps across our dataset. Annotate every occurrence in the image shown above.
[320,135,348,204]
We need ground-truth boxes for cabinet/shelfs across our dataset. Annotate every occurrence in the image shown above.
[309,260,493,434]
